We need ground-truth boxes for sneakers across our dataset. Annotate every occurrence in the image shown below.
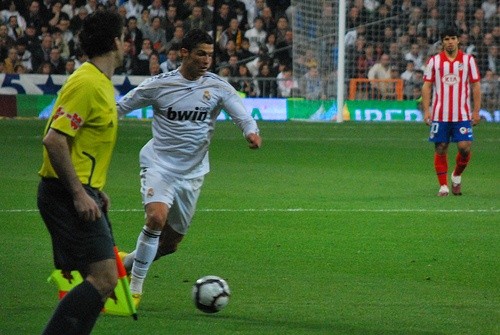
[131,294,142,311]
[437,186,449,196]
[118,251,131,284]
[451,173,462,195]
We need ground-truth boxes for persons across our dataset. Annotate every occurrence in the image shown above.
[0,0,500,100]
[36,9,128,335]
[117,27,262,309]
[421,31,483,197]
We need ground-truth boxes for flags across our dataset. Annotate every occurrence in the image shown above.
[48,246,137,317]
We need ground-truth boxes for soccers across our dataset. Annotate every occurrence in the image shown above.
[192,275,230,314]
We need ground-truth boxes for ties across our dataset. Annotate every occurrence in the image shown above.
[13,28,18,39]
